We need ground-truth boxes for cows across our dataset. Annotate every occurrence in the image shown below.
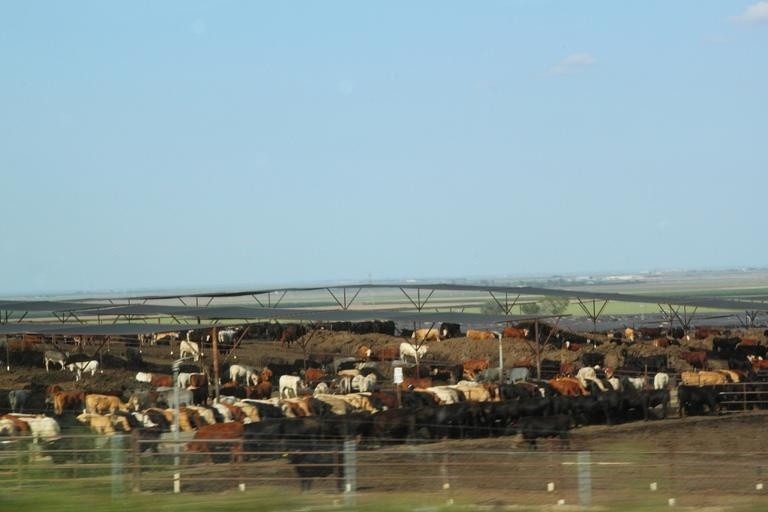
[0,320,768,465]
[280,448,334,494]
